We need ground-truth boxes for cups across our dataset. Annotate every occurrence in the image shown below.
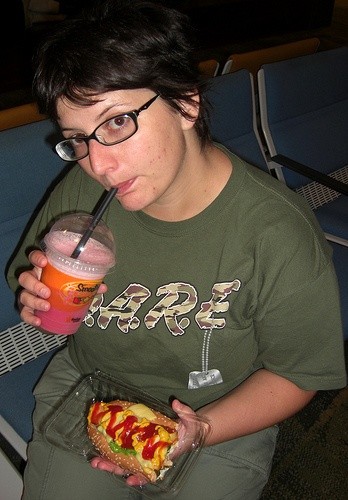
[31,212,120,334]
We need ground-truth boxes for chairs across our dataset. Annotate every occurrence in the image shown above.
[0,37,348,500]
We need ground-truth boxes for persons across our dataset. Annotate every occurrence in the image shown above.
[6,2,347,500]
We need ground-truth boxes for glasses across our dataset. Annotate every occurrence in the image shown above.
[54,93,160,162]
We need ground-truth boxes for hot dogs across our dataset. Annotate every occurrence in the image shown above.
[86,399,179,481]
